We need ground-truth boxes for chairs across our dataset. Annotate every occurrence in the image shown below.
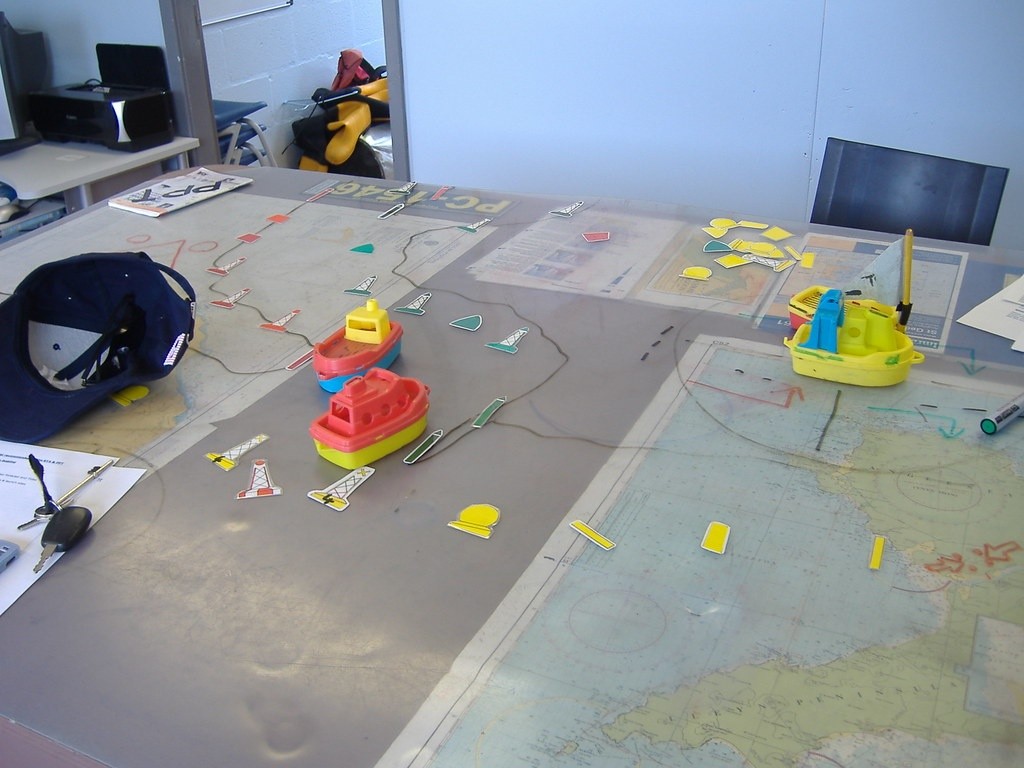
[809,136,1010,247]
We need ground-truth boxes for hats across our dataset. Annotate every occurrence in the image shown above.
[0,252,195,443]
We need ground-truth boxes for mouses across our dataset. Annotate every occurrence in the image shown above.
[0,203,30,224]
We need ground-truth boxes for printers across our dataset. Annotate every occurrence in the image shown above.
[22,82,174,153]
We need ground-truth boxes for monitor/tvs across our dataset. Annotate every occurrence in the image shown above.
[0,11,44,156]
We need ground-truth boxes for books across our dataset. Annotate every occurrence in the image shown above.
[107,165,253,218]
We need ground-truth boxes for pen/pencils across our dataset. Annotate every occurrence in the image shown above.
[56,458,114,504]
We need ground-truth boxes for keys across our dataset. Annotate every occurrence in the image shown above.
[17,451,114,574]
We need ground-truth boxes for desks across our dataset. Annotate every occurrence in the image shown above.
[0,163,1024,768]
[0,136,200,215]
